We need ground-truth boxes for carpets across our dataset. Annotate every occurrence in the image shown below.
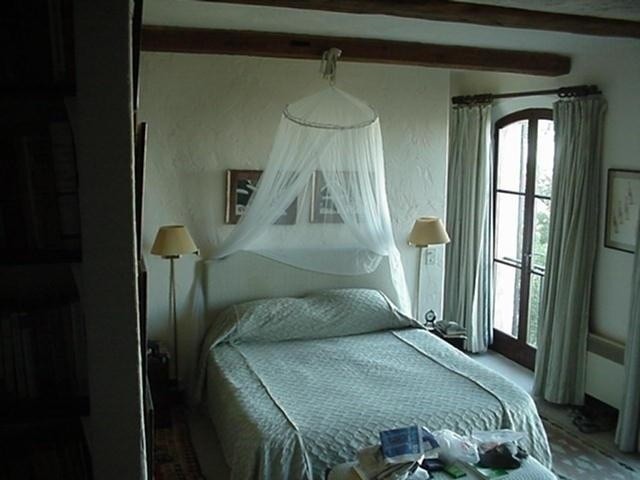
[535,409,637,480]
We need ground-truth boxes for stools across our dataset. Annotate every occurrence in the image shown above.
[329,453,561,480]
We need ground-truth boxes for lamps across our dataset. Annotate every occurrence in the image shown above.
[150,225,200,392]
[408,216,451,323]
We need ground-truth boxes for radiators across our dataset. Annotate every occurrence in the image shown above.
[585,332,626,412]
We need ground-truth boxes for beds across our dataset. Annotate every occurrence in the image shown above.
[195,244,539,478]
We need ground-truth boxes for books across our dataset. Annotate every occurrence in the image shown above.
[435,320,467,340]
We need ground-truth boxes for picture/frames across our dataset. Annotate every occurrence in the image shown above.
[604,168,640,255]
[223,168,300,227]
[309,169,365,225]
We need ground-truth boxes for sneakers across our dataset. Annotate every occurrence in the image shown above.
[574,414,612,433]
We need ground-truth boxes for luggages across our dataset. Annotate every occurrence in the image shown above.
[146,338,173,428]
